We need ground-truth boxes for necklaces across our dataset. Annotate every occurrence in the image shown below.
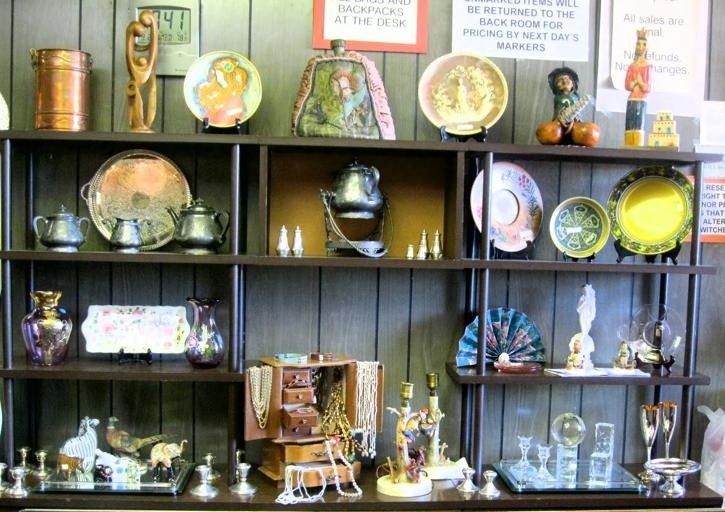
[249,361,380,505]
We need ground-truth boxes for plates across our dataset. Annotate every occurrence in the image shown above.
[183,51,263,128]
[80,304,193,354]
[82,149,193,248]
[551,197,611,258]
[470,160,543,253]
[418,51,509,137]
[607,165,696,255]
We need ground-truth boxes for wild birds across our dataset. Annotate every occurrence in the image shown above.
[105,416,179,458]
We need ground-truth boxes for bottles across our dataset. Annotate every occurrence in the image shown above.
[276,225,303,258]
[415,229,444,259]
[19,289,72,366]
[183,296,224,366]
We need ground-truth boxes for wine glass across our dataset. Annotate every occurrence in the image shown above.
[640,404,661,480]
[659,401,678,457]
[643,456,702,495]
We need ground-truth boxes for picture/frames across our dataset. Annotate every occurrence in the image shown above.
[311,0,430,57]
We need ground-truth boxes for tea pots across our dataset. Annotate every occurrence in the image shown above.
[162,197,230,256]
[32,203,92,254]
[98,213,151,253]
[324,156,384,213]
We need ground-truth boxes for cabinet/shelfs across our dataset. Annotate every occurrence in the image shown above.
[0,124,723,511]
